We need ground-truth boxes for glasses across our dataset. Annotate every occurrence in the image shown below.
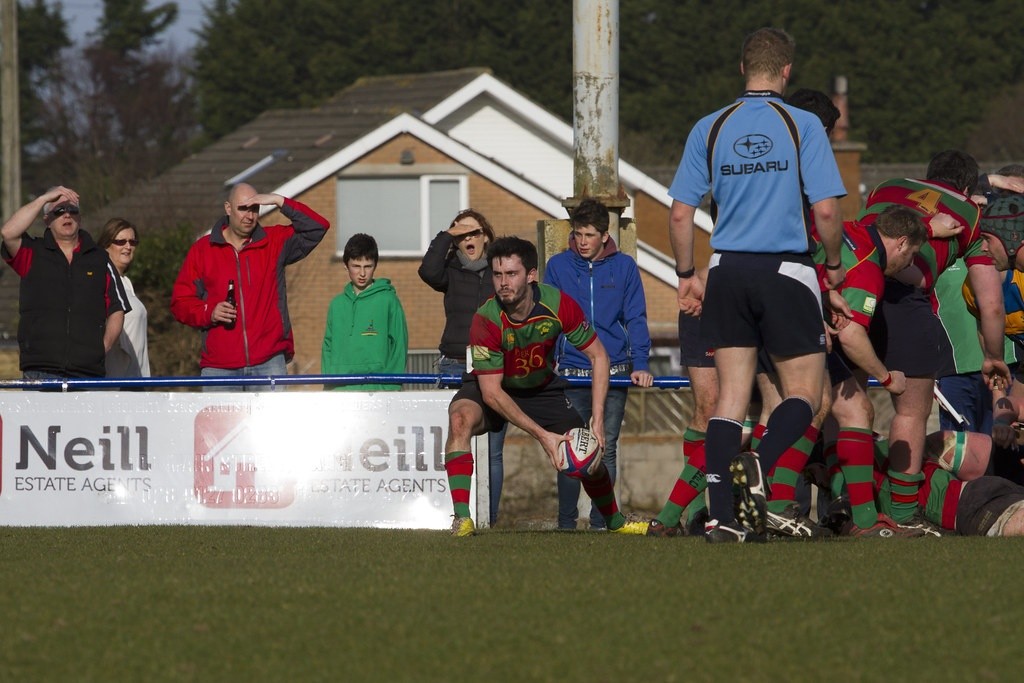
[111,239,139,246]
[44,206,79,216]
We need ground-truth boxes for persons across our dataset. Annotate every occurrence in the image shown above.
[418,210,509,528]
[0,185,152,393]
[321,233,408,392]
[653,89,1024,538]
[170,184,331,393]
[653,27,846,544]
[548,201,653,532]
[444,236,666,538]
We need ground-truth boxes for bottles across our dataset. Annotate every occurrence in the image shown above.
[224,278,236,330]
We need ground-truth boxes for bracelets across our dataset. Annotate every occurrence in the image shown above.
[878,372,893,388]
[824,256,842,270]
[673,263,695,279]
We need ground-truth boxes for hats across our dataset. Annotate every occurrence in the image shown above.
[979,196,1024,256]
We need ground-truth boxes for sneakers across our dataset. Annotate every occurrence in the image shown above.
[648,518,678,538]
[843,515,925,539]
[766,502,832,538]
[451,514,474,536]
[704,519,750,543]
[610,511,650,536]
[898,517,957,536]
[728,452,769,536]
